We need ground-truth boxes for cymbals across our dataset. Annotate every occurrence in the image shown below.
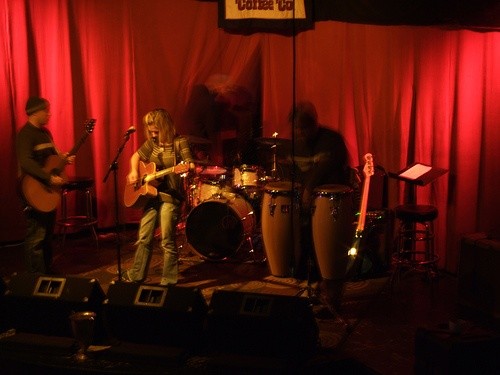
[179,134,212,144]
[192,159,221,166]
[255,138,292,146]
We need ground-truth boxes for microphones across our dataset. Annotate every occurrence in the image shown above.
[125,126,136,135]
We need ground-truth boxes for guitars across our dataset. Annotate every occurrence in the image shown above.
[341,154,386,278]
[123,160,190,210]
[21,118,96,213]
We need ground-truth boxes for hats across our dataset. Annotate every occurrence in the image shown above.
[25,96,50,115]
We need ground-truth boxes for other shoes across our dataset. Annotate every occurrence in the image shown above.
[111,272,132,284]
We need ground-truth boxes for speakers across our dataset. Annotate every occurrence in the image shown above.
[0,272,322,356]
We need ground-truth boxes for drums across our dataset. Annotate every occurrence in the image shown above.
[185,191,256,261]
[310,184,357,280]
[188,178,222,208]
[260,181,305,277]
[231,165,263,190]
[195,166,228,189]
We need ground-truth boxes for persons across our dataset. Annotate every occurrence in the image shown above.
[110,109,195,286]
[17,95,77,287]
[274,104,351,209]
